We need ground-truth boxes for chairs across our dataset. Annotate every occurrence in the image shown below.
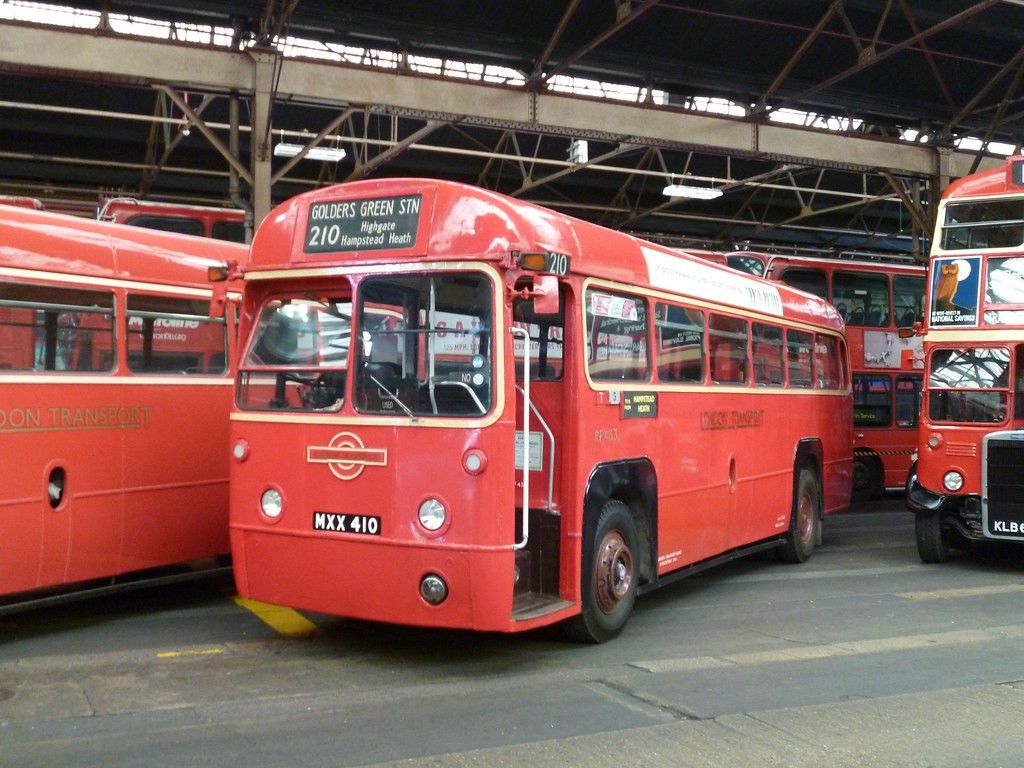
[835,301,924,327]
[315,363,837,414]
[142,358,225,374]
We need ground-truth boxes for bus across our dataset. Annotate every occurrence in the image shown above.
[0,155,1024,646]
